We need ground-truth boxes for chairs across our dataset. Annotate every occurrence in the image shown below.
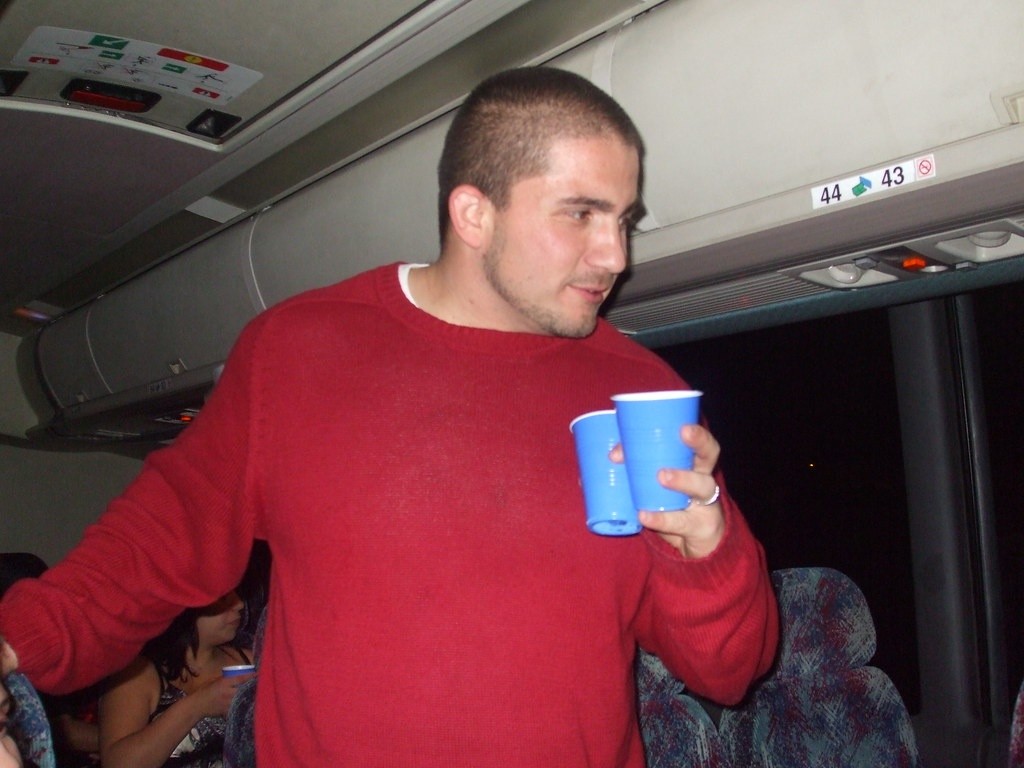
[1,553,920,767]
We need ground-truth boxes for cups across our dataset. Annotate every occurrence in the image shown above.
[220,664,257,677]
[612,391,704,512]
[569,409,645,535]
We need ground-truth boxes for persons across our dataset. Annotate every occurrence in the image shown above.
[0,577,257,767]
[0,65,780,768]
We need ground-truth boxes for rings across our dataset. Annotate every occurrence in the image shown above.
[691,485,721,508]
